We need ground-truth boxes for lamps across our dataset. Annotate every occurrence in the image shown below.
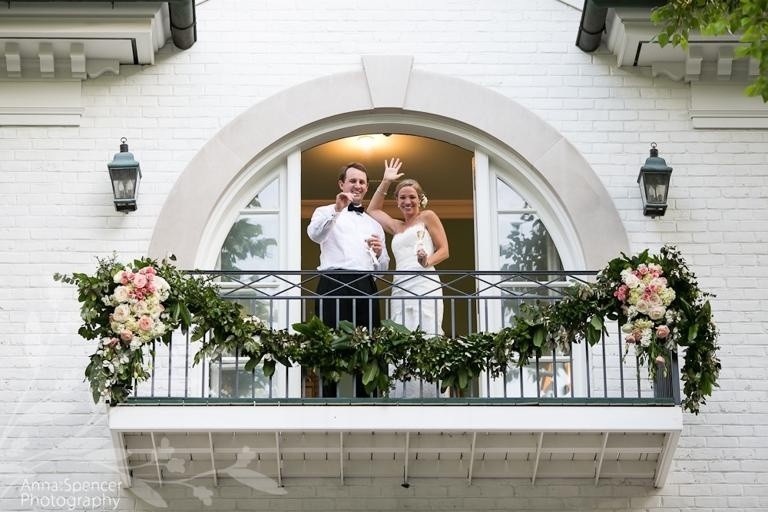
[106,137,143,211]
[636,142,672,219]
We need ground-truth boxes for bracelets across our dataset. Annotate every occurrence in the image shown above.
[376,187,388,196]
[424,262,429,268]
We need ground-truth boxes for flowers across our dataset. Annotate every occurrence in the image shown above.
[49,245,727,419]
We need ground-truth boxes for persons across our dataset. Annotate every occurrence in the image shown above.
[306,161,391,398]
[366,157,449,398]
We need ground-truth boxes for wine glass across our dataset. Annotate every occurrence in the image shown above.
[417,222,426,258]
[364,232,374,270]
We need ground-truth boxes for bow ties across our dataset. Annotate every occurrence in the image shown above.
[348,204,364,213]
